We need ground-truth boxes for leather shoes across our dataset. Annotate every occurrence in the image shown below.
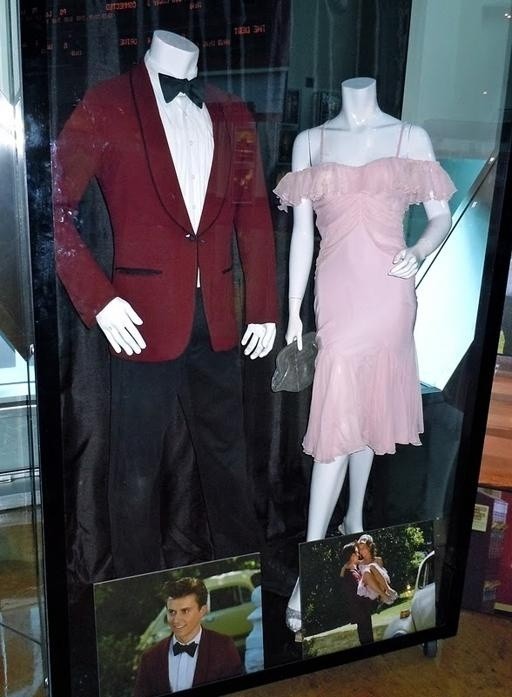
[381,590,398,604]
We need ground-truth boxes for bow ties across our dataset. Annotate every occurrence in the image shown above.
[158,73,205,108]
[173,642,198,657]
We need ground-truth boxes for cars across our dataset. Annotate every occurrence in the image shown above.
[381,552,436,642]
[129,563,262,680]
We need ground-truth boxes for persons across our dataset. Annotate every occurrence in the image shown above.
[48,27,306,647]
[281,76,457,632]
[341,532,399,606]
[132,573,245,697]
[339,540,376,642]
